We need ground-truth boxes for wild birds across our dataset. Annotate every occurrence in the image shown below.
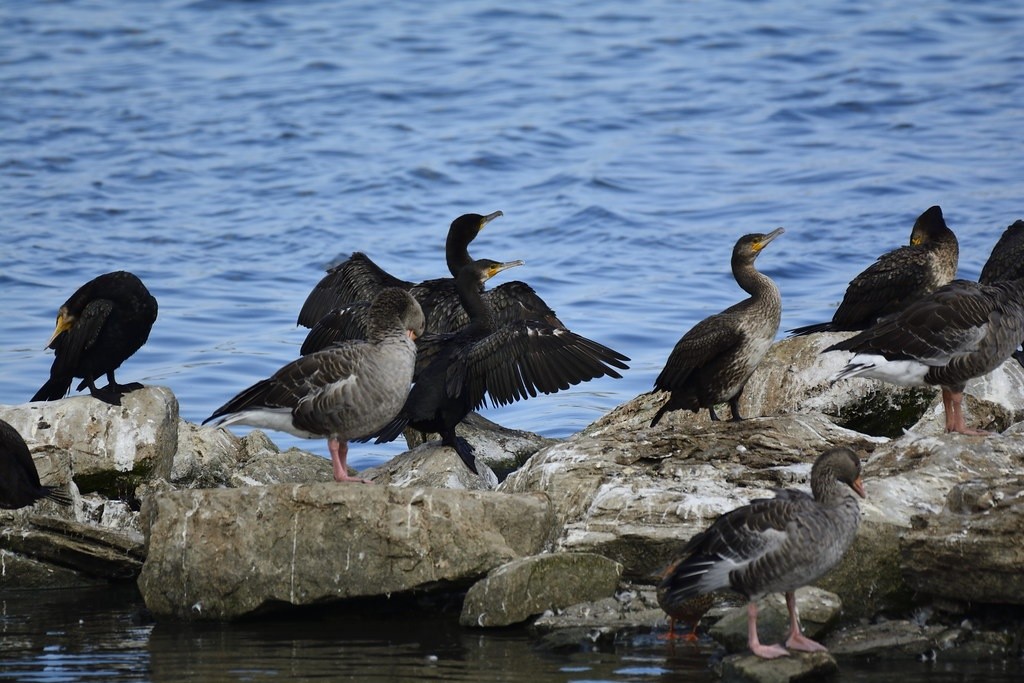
[0,419,75,510]
[650,205,1023,439]
[198,211,631,487]
[655,446,866,662]
[29,271,158,408]
